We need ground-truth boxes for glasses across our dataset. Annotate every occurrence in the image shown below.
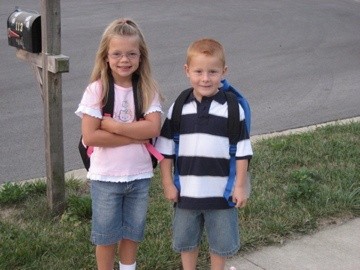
[108,52,141,58]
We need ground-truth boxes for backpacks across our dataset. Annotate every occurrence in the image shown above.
[78,75,164,172]
[173,79,251,207]
